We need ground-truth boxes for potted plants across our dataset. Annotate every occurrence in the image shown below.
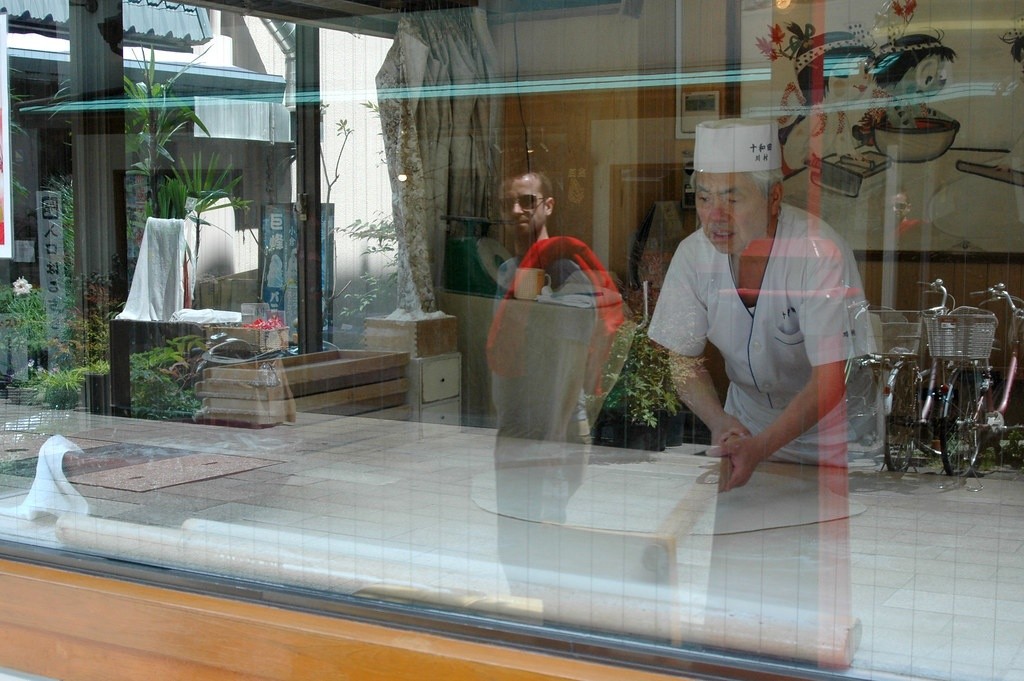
[594,315,693,454]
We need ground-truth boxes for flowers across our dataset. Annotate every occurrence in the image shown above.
[1,280,84,387]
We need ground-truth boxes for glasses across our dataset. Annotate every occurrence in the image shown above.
[497,194,546,211]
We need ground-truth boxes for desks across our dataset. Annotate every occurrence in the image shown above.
[0,397,1022,679]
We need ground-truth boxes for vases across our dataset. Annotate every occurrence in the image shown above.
[5,389,45,404]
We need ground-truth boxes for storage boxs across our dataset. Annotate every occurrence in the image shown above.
[361,313,460,359]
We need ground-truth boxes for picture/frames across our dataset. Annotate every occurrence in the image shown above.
[722,0,1023,265]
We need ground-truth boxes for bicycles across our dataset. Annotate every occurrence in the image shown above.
[843,275,1024,486]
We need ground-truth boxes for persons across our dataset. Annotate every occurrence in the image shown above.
[486,166,622,595]
[646,112,878,472]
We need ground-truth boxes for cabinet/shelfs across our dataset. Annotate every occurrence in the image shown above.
[407,351,465,440]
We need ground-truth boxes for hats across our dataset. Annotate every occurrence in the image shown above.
[693,117,784,173]
